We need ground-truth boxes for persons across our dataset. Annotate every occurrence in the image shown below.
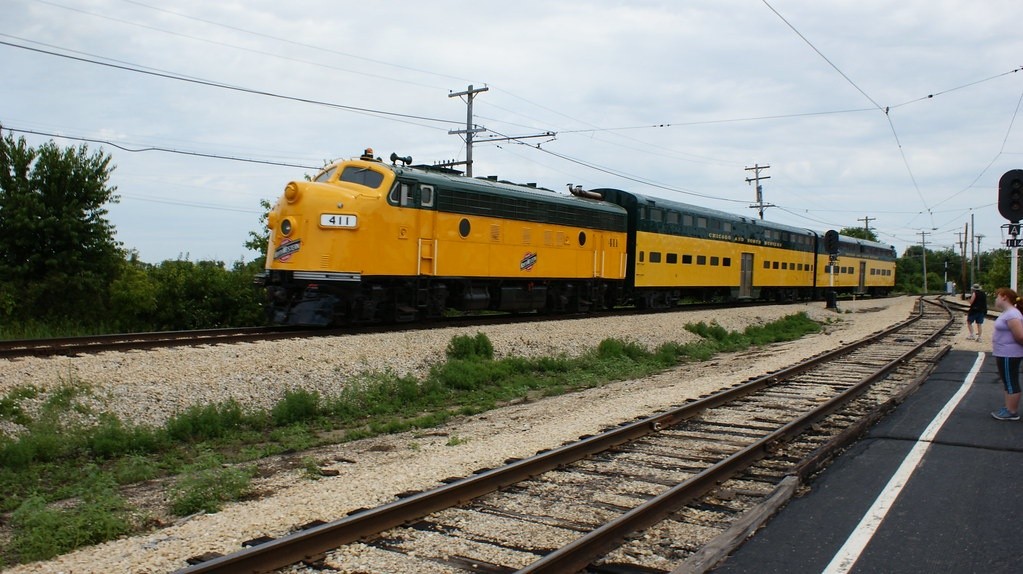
[991,288,1023,420]
[967,284,987,343]
[952,280,958,296]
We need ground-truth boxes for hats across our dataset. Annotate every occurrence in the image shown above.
[971,284,982,290]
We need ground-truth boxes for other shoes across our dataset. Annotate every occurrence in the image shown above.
[977,337,982,343]
[966,334,976,340]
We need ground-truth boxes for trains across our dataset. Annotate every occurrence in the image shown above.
[244,149,901,330]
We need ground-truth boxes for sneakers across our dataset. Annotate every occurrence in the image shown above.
[991,407,1021,421]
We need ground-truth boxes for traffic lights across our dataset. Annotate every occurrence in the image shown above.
[998,168,1023,223]
